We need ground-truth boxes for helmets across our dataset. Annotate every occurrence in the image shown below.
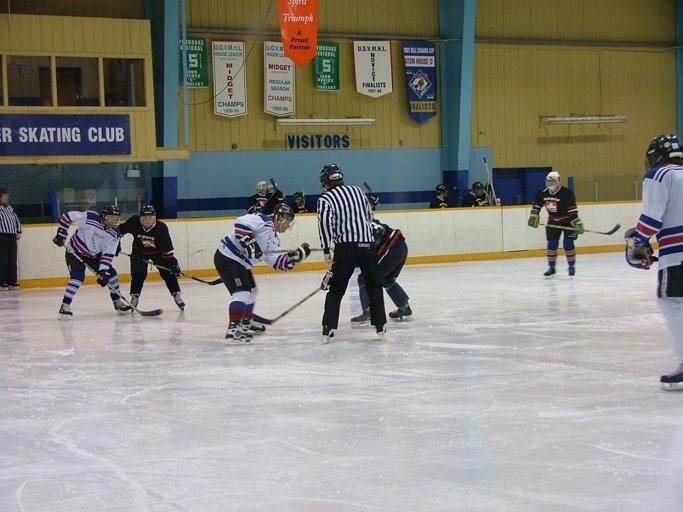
[100,201,121,215]
[472,182,485,189]
[436,184,448,194]
[320,164,343,188]
[646,134,683,167]
[545,171,561,193]
[272,203,294,222]
[256,181,267,193]
[140,205,156,216]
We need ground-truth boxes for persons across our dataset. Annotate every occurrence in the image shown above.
[51,200,133,315]
[429,184,451,208]
[0,189,21,288]
[214,161,413,341]
[626,134,683,385]
[528,172,584,276]
[114,206,186,309]
[460,182,488,208]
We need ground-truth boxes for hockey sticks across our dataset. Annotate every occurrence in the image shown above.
[119,249,222,285]
[63,240,163,318]
[250,287,323,325]
[540,222,621,234]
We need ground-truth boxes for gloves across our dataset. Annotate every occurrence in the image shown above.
[166,256,180,278]
[528,208,540,228]
[625,227,654,269]
[97,269,111,287]
[52,226,68,247]
[284,242,310,270]
[240,233,262,259]
[321,270,334,290]
[571,216,583,235]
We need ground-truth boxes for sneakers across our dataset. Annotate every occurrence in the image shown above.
[351,311,371,321]
[0,280,19,286]
[226,318,265,340]
[661,364,683,383]
[171,290,185,307]
[323,325,334,337]
[110,293,138,311]
[569,267,574,275]
[389,306,412,318]
[59,299,73,315]
[544,267,555,276]
